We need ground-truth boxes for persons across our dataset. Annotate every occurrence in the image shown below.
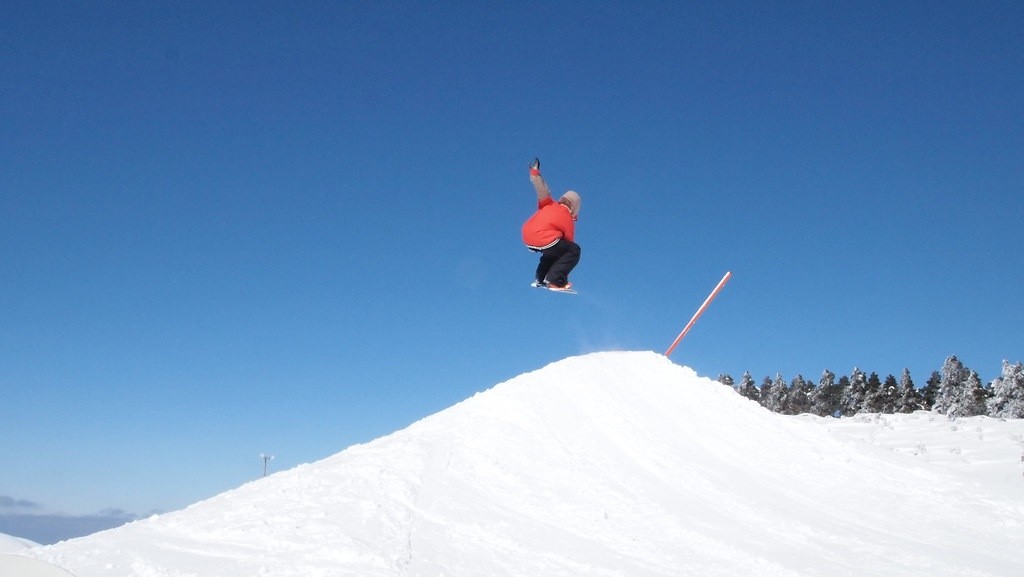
[521,156,582,291]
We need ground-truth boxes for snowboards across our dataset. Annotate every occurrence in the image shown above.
[530,282,578,295]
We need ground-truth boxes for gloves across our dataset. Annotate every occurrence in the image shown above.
[527,157,540,175]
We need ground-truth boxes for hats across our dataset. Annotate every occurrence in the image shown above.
[557,191,580,217]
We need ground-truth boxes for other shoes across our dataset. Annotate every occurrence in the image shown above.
[535,278,572,290]
